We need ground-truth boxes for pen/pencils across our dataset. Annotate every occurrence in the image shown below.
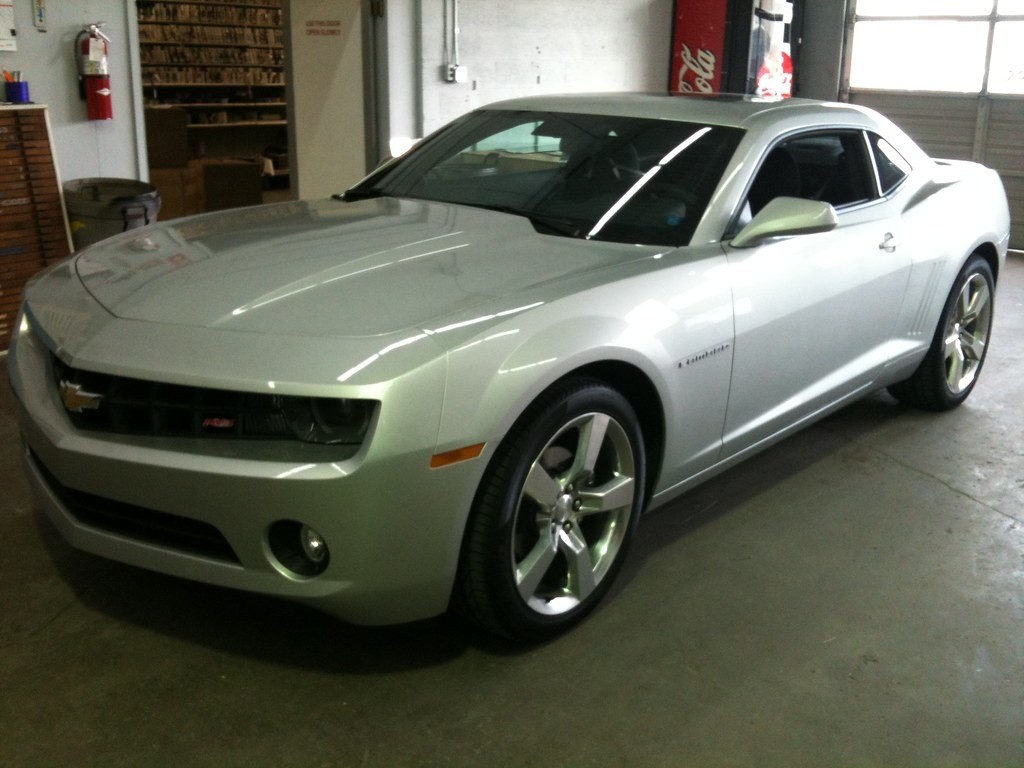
[3,70,23,82]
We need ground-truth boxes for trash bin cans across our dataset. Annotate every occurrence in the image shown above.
[268,151,289,190]
[60,176,162,253]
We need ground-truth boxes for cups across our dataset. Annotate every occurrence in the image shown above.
[218,112,227,123]
[6,82,29,104]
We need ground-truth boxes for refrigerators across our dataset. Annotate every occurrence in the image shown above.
[669,0,801,98]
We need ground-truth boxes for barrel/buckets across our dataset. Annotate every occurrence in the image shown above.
[62,177,161,251]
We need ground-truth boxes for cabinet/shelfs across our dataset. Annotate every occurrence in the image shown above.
[138,0,289,129]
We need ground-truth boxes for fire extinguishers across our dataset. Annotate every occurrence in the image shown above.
[75,21,115,122]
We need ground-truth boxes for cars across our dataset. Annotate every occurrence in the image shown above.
[8,89,1011,653]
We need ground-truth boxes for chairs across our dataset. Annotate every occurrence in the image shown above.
[748,146,801,206]
[786,141,843,201]
